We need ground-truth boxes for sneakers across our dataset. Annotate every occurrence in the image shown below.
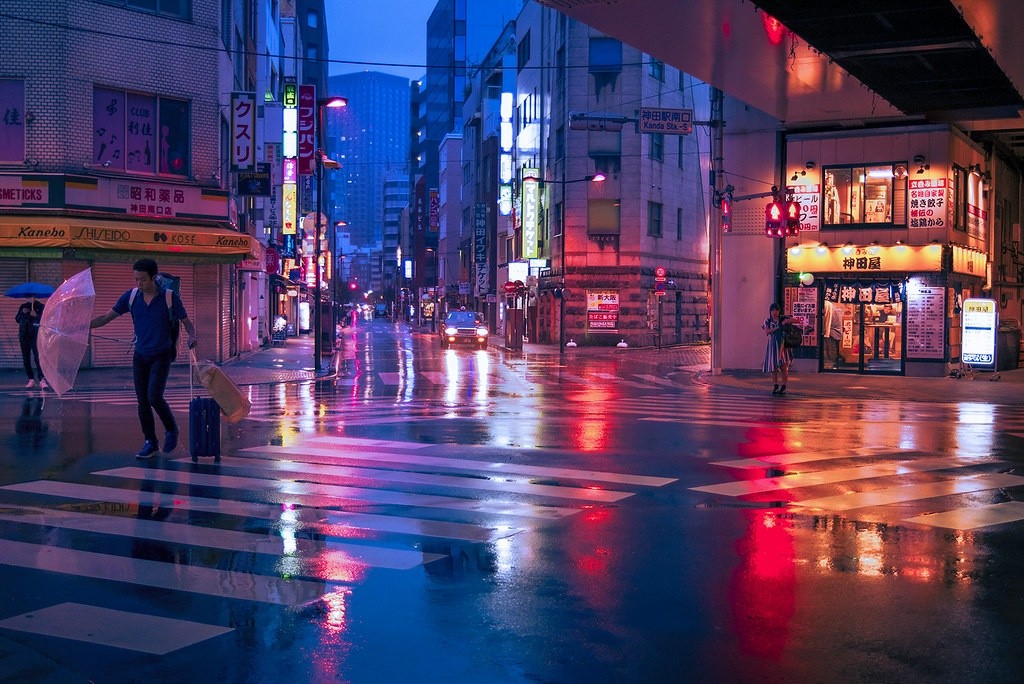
[163,428,179,454]
[135,439,159,459]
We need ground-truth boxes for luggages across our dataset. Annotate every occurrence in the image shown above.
[189,343,221,463]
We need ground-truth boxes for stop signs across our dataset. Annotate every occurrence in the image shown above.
[504,282,515,292]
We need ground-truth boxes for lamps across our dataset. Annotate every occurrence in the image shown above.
[791,242,799,247]
[947,240,986,254]
[791,171,806,180]
[26,112,33,122]
[916,164,930,174]
[843,241,853,246]
[869,241,878,246]
[931,240,939,244]
[818,242,828,247]
[895,240,904,244]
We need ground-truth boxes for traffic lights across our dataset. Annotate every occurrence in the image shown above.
[765,201,801,238]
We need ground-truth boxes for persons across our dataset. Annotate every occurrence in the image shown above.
[15,297,47,389]
[345,306,352,325]
[824,301,845,369]
[761,303,798,395]
[868,304,896,354]
[404,303,410,322]
[63,258,197,459]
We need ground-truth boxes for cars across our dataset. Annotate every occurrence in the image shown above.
[374,303,388,318]
[439,305,489,350]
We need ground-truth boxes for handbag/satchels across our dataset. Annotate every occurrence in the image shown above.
[785,324,802,348]
[190,348,252,424]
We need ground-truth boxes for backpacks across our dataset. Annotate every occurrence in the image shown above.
[128,272,181,361]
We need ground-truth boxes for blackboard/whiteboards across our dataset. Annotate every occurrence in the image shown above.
[270,315,287,341]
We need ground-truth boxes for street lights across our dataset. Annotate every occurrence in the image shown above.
[314,96,350,371]
[333,220,348,346]
[522,172,606,354]
[426,247,437,333]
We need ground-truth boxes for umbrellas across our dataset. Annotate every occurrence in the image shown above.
[33,267,139,396]
[4,282,55,298]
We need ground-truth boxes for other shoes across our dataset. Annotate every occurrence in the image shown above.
[780,387,786,395]
[25,379,36,388]
[772,387,779,395]
[39,377,48,388]
